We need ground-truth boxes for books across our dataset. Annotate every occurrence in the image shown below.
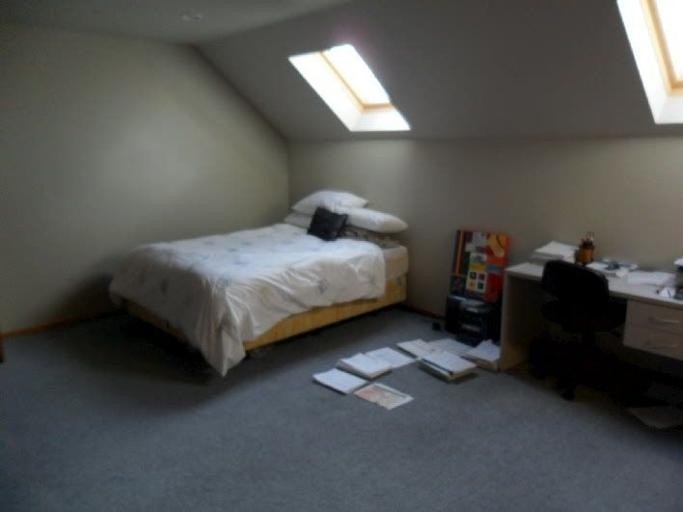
[310,325,502,412]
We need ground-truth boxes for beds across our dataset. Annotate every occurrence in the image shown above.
[107,218,408,375]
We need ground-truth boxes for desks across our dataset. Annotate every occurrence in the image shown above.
[497,262,683,371]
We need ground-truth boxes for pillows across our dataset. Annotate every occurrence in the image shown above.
[283,189,410,246]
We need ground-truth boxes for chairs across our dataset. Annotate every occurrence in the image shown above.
[521,256,621,400]
